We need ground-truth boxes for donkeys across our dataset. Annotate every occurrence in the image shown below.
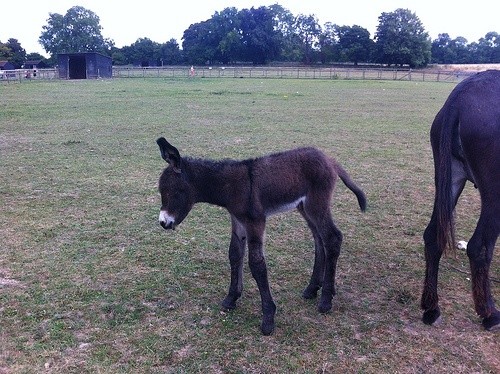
[419,68,500,332]
[152,133,367,336]
